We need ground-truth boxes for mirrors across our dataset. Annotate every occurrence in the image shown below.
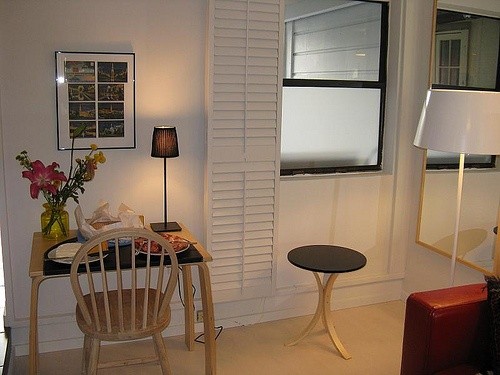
[415,0,500,281]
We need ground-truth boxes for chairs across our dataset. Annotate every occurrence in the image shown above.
[70,227,178,375]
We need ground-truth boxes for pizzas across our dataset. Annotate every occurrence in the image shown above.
[135,233,188,253]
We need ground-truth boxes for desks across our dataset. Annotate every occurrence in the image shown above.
[27,224,219,375]
[286,244,366,360]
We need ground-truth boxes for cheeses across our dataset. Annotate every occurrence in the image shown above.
[56,243,81,258]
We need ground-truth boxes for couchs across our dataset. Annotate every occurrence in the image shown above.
[400,281,500,375]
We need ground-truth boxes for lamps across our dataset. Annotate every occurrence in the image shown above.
[150,125,182,232]
[413,82,500,290]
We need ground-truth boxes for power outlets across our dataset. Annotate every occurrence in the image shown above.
[196,310,205,322]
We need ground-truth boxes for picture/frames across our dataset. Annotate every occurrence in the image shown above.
[54,50,138,150]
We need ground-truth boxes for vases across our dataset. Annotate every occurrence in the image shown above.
[41,202,71,240]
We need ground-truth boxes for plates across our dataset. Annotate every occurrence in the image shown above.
[135,235,190,256]
[47,247,108,265]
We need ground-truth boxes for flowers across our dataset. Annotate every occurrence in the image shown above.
[15,125,105,224]
